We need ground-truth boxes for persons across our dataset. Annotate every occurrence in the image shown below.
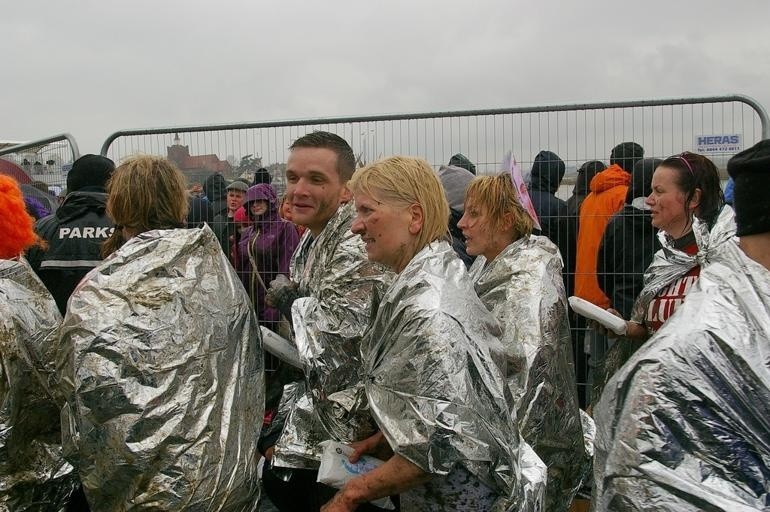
[21,154,124,320]
[54,155,266,512]
[535,140,737,410]
[186,167,305,327]
[593,139,770,511]
[265,131,373,512]
[437,153,478,271]
[457,173,587,512]
[0,176,69,512]
[320,156,548,512]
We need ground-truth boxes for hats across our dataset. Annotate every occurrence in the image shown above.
[610,142,643,172]
[226,181,249,192]
[67,154,115,194]
[726,138,769,238]
